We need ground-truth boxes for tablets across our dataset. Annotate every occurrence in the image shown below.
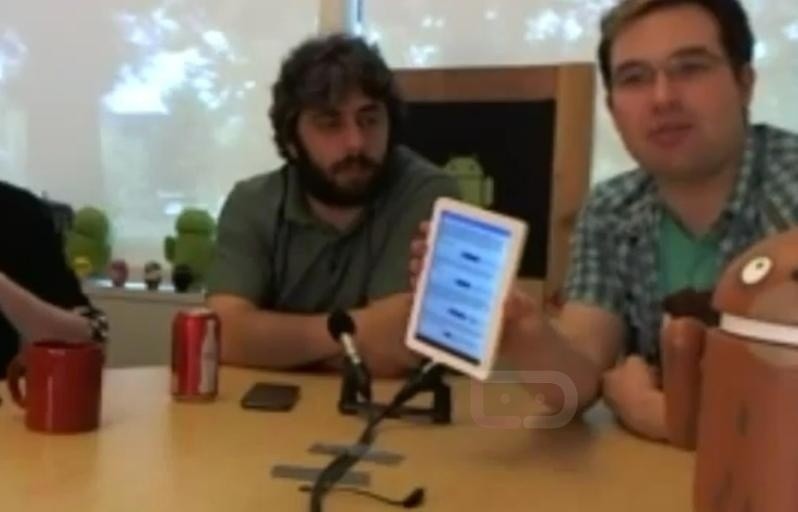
[401,196,530,381]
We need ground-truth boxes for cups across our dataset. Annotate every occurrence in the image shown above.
[8,339,103,432]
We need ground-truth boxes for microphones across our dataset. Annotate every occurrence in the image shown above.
[326,306,373,399]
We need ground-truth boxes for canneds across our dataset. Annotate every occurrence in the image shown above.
[169,308,220,401]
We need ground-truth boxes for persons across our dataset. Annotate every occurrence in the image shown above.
[204,35,463,376]
[0,182,110,377]
[409,0,798,446]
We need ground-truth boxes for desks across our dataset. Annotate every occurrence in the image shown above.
[1,365,694,511]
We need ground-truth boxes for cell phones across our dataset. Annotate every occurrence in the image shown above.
[241,384,295,410]
